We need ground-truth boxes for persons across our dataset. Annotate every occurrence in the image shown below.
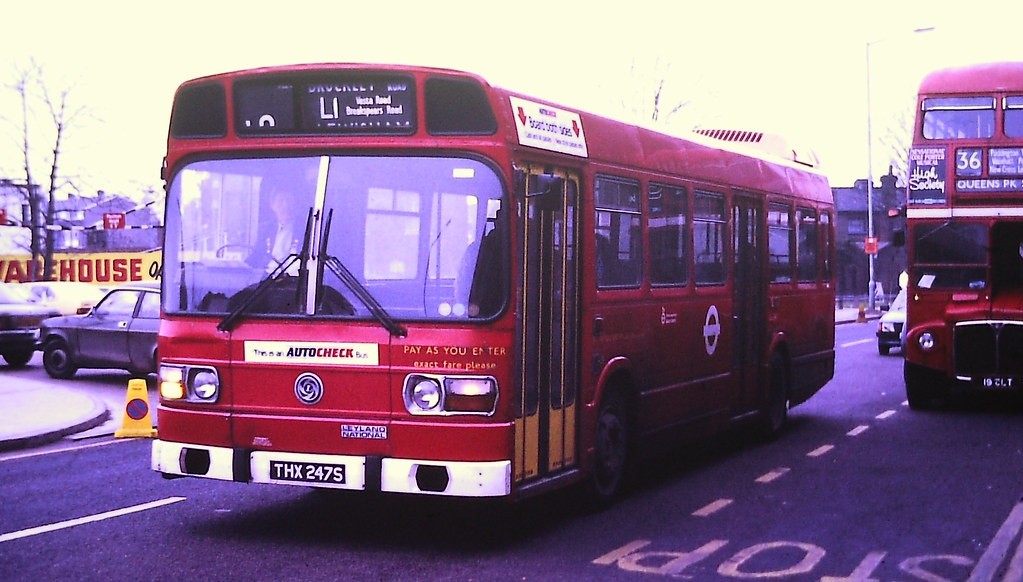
[241,185,314,277]
[455,205,620,324]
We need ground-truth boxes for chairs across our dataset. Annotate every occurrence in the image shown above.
[584,232,822,289]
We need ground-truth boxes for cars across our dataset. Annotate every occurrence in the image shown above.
[875,290,908,355]
[0,288,63,367]
[40,280,164,381]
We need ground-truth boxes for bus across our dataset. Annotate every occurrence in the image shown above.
[900,60,1021,410]
[150,62,839,510]
